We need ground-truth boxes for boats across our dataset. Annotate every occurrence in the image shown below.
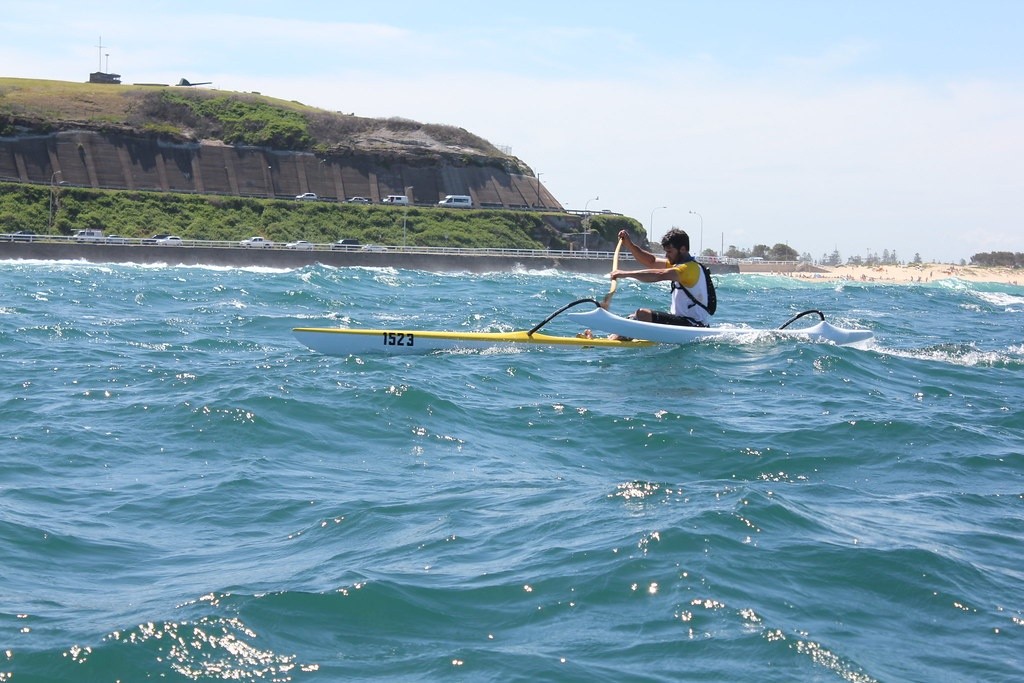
[569,309,873,345]
[293,299,680,358]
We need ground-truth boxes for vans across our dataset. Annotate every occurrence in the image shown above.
[438,194,472,208]
[331,238,359,250]
[383,195,408,205]
[72,229,103,243]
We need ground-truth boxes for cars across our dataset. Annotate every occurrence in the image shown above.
[361,244,388,252]
[348,197,369,204]
[286,240,312,250]
[105,235,129,244]
[295,193,317,202]
[3,230,37,241]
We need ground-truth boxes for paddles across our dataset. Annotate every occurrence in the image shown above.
[599,237,624,310]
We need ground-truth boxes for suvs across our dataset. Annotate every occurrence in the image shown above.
[142,234,172,245]
[156,236,183,246]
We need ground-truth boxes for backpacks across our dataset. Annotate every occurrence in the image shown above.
[679,260,717,315]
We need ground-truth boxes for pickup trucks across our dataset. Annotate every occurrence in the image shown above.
[239,237,275,249]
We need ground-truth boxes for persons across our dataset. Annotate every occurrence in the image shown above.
[610,229,709,328]
